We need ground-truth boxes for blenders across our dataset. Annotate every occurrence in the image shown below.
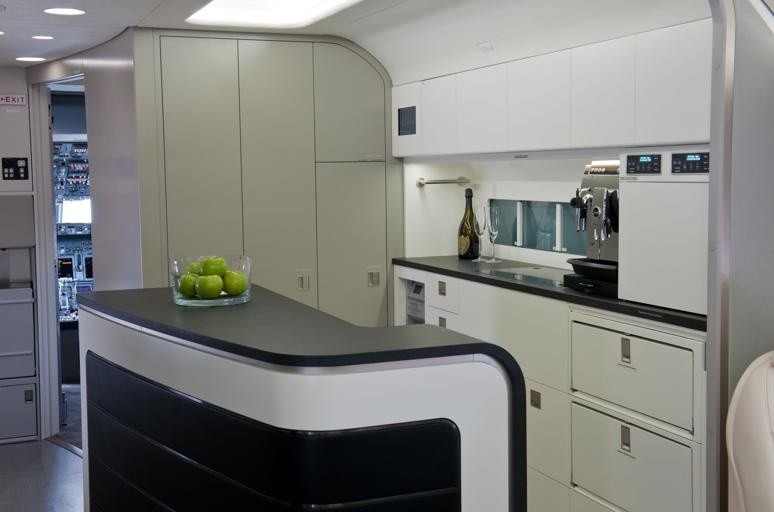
[565,159,619,282]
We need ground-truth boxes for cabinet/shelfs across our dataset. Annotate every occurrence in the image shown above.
[2,287,37,439]
[459,279,571,489]
[313,35,389,327]
[154,29,243,328]
[388,17,711,163]
[571,305,705,511]
[236,32,316,304]
[423,270,459,331]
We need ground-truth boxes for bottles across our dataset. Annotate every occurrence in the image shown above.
[457,187,480,261]
[511,200,537,248]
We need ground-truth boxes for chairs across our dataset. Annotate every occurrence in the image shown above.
[724,351,773,512]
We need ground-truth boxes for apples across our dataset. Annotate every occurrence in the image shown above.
[186,262,202,276]
[223,270,249,297]
[179,274,196,298]
[203,256,229,277]
[195,274,224,299]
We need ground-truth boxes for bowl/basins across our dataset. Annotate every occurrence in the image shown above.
[168,253,253,307]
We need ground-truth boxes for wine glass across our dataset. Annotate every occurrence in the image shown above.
[537,216,552,251]
[485,205,503,265]
[470,206,490,262]
